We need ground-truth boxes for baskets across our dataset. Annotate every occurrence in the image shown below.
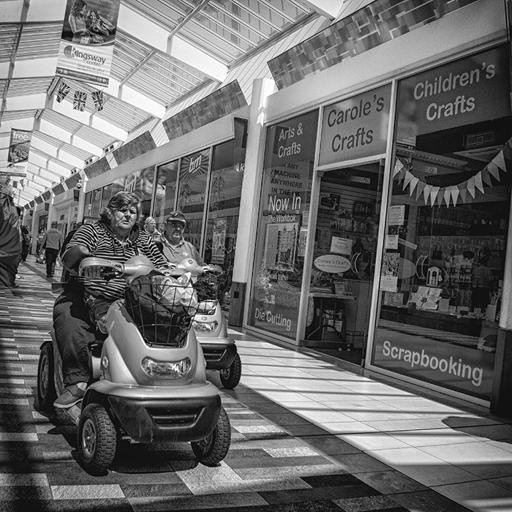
[125,285,200,348]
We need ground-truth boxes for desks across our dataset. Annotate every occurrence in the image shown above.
[254,284,355,351]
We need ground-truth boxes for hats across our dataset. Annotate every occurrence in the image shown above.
[167,215,186,224]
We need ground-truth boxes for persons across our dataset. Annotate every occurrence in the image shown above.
[142,216,161,240]
[52,190,203,410]
[39,221,63,278]
[154,210,216,271]
[59,222,83,281]
[36,226,44,257]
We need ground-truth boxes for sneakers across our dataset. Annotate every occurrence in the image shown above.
[47,274,55,277]
[53,384,86,409]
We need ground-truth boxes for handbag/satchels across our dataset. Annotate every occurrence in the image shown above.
[194,273,228,301]
[126,270,189,343]
